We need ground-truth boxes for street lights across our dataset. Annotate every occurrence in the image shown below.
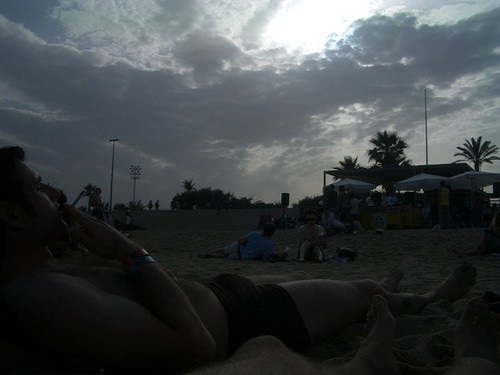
[108,138,119,217]
[129,164,141,227]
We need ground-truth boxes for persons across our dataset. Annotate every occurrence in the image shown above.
[91,187,105,221]
[297,209,328,263]
[0,145,500,375]
[198,223,276,261]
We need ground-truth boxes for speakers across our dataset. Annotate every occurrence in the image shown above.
[282,193,289,206]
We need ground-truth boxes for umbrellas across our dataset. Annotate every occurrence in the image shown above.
[393,173,449,193]
[325,177,375,193]
[448,170,500,190]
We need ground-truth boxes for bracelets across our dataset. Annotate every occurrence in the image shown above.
[119,247,154,271]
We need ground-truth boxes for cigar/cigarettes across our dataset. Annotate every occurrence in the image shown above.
[72,190,85,207]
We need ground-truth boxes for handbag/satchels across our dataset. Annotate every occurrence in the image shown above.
[336,247,358,262]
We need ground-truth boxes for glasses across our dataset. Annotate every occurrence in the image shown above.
[306,217,315,221]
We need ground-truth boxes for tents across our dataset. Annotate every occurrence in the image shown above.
[324,162,475,190]
